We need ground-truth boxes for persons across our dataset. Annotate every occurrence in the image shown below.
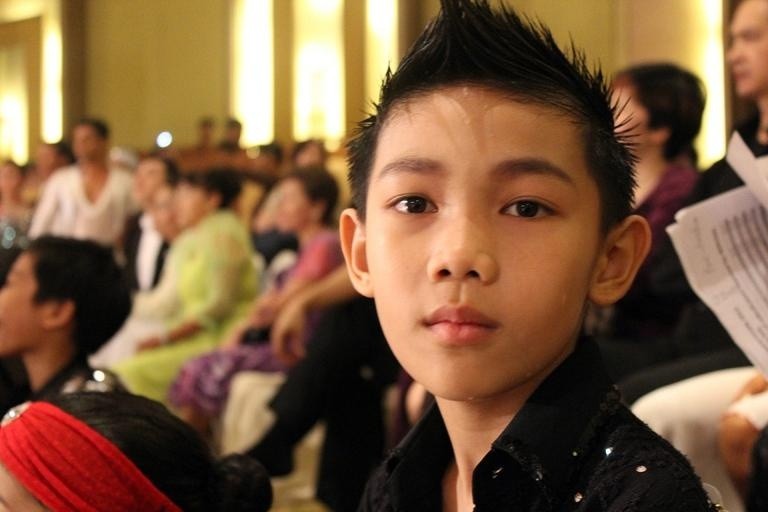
[0,0,767,512]
